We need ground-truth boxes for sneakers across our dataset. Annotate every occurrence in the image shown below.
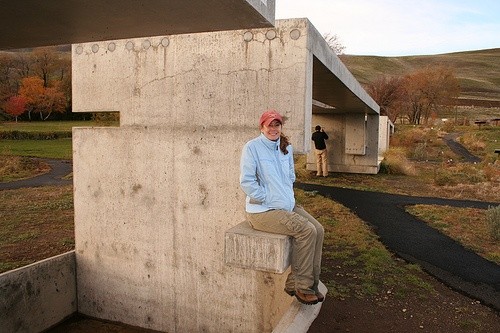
[283,284,318,304]
[316,290,324,302]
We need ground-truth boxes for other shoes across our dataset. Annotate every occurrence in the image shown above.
[317,176,320,178]
[323,176,327,178]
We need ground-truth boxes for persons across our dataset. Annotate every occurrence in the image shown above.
[239,110,324,305]
[312,125,330,177]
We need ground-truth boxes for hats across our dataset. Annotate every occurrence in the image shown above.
[260,111,283,128]
